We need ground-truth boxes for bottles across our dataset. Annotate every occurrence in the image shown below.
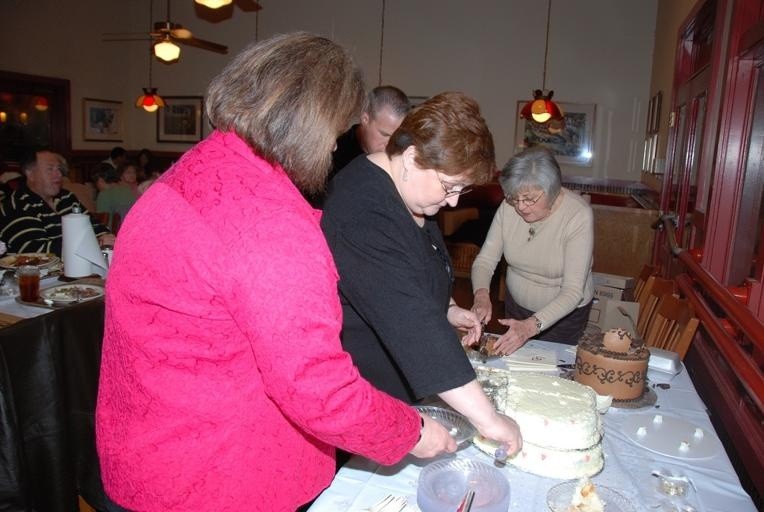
[101,245,114,269]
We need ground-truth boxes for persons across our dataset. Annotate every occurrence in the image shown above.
[469,145,594,361]
[325,85,410,188]
[0,145,181,262]
[96,32,461,511]
[320,89,524,474]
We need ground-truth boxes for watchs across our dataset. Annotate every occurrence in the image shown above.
[533,316,542,334]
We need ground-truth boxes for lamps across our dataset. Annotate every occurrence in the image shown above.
[195,0,233,9]
[132,0,169,115]
[150,37,182,63]
[517,1,567,128]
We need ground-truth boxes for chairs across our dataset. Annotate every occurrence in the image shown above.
[438,200,495,283]
[62,159,137,237]
[626,261,701,362]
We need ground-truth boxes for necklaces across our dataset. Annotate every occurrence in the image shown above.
[521,218,543,243]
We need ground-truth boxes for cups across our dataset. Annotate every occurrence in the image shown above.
[15,265,43,302]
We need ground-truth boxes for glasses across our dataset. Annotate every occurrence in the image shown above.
[438,167,476,196]
[507,194,547,205]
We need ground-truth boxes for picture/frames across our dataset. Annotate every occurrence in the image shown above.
[154,94,204,145]
[80,97,124,144]
[510,96,596,169]
[403,94,429,112]
[640,89,664,175]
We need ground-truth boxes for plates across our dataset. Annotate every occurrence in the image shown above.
[544,479,634,511]
[39,283,105,303]
[407,406,477,451]
[1,252,60,271]
[415,458,509,512]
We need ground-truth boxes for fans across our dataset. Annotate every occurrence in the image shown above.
[189,0,264,24]
[99,0,229,57]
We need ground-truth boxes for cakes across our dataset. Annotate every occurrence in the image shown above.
[573,326,651,401]
[478,333,500,356]
[471,371,605,481]
[566,484,604,511]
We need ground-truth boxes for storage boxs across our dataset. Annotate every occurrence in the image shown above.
[589,270,637,307]
[578,292,641,341]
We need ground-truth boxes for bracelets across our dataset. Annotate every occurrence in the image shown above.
[448,302,458,308]
[412,416,425,446]
